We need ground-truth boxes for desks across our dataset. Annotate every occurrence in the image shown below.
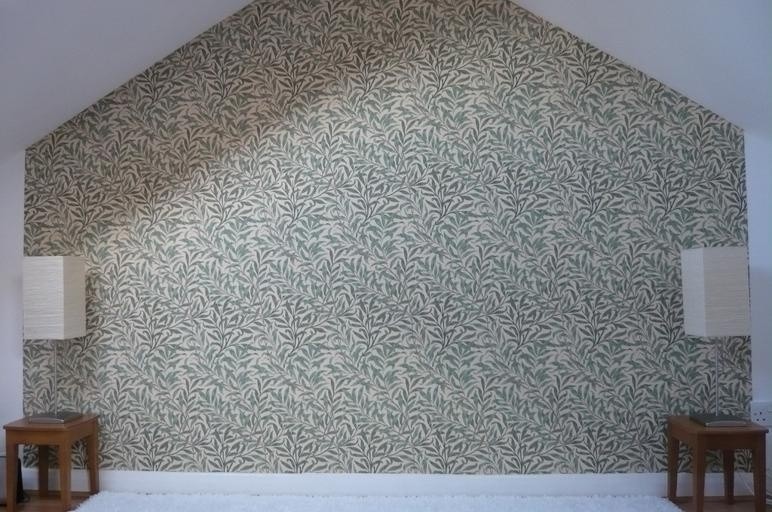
[3,415,100,512]
[667,414,769,512]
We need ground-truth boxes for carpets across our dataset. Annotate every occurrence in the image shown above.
[68,490,685,512]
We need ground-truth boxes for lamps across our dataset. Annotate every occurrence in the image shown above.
[680,247,751,428]
[22,256,87,423]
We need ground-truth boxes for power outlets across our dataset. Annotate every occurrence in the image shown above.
[750,402,772,427]
